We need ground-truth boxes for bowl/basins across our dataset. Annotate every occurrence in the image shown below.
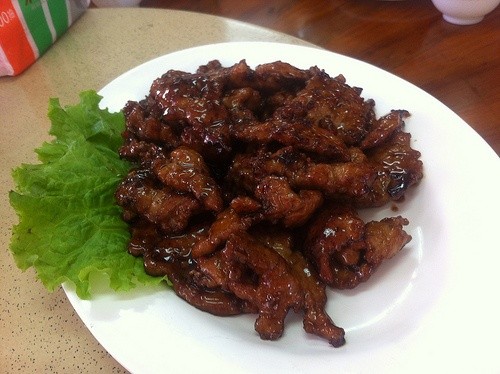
[432,0,500,25]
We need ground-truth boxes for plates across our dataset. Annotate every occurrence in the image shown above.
[60,41,500,373]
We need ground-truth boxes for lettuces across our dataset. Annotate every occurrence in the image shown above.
[7,89,174,299]
